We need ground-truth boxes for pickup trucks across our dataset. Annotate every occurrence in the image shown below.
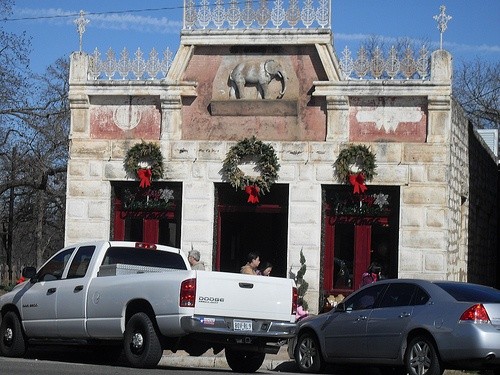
[0,240,300,373]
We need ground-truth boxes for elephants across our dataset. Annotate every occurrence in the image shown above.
[229,58,287,100]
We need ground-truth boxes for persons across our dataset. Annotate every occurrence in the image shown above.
[360,263,382,288]
[256,262,272,277]
[295,299,309,321]
[239,253,260,275]
[188,250,205,271]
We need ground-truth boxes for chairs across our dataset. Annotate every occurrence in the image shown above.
[64,253,109,275]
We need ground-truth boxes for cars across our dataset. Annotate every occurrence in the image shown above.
[287,278,500,375]
